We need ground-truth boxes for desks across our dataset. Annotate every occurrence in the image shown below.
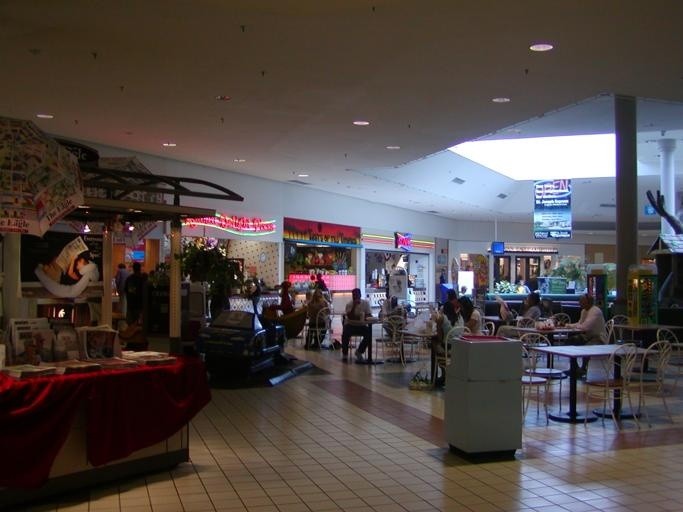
[530,342,660,425]
[0,354,215,506]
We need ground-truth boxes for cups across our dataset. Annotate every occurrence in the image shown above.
[359,311,364,321]
[425,321,432,332]
[338,269,347,275]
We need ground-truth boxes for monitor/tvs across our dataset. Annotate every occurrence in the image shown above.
[491,242,504,254]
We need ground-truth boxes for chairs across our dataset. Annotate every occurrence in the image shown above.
[299,306,681,388]
[517,333,566,413]
[583,344,642,432]
[627,340,676,429]
[516,347,550,428]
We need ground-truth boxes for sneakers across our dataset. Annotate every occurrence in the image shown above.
[342,355,348,361]
[354,350,365,361]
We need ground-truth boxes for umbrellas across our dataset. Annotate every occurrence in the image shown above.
[1,115,85,240]
[56,157,167,246]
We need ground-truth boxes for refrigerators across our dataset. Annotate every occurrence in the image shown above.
[586,264,608,322]
[625,264,658,326]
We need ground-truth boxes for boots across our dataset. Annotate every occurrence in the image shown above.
[314,334,324,352]
[304,335,313,351]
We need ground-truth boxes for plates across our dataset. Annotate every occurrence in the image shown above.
[365,318,373,321]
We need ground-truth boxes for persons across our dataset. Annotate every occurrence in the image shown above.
[435,289,460,387]
[440,269,448,284]
[341,288,372,361]
[209,254,239,321]
[113,261,156,327]
[557,293,606,378]
[244,272,294,297]
[458,286,553,335]
[540,259,551,276]
[304,272,330,349]
[378,294,407,335]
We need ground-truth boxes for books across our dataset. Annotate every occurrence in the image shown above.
[1,317,177,378]
